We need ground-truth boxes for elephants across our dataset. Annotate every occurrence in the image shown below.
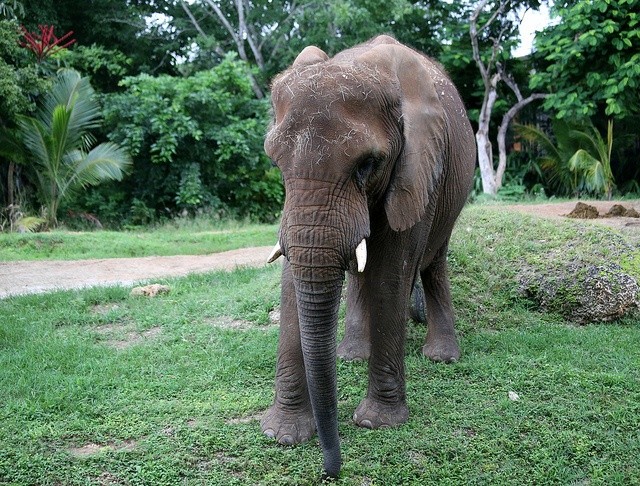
[260,34,478,478]
